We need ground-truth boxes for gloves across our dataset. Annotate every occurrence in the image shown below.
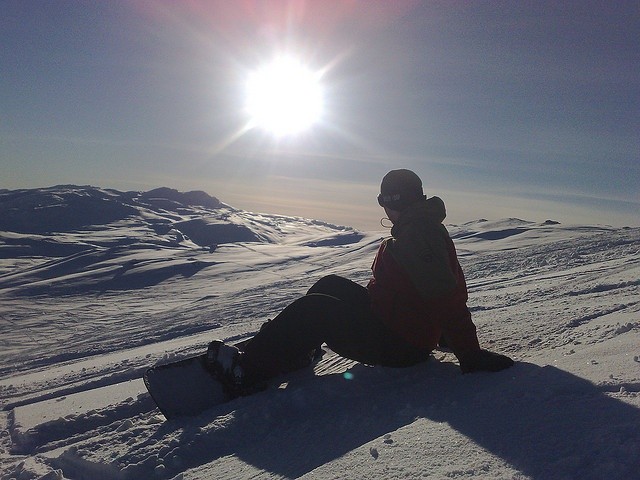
[460,349,514,375]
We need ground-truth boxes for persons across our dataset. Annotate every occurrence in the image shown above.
[205,169,515,400]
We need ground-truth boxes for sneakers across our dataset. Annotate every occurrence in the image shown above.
[207,341,262,396]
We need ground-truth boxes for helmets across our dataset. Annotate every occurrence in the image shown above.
[377,169,424,212]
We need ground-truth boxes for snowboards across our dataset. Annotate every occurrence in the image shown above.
[143,335,320,426]
